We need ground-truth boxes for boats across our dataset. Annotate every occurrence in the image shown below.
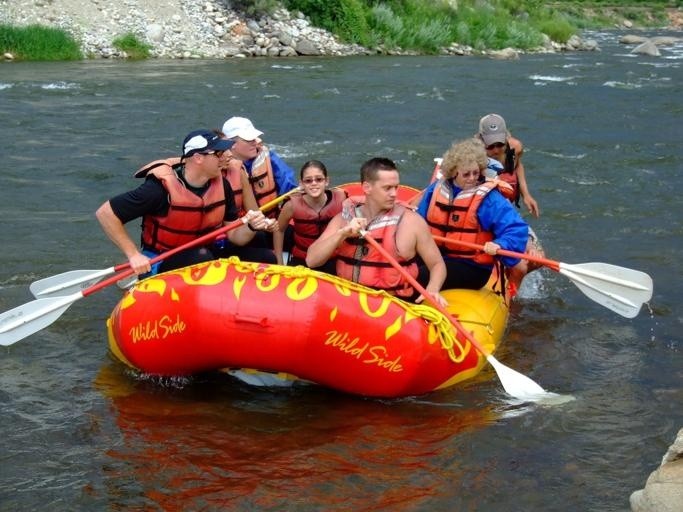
[109,182,519,398]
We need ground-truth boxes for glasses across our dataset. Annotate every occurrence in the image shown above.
[198,149,224,158]
[486,142,505,150]
[302,178,326,184]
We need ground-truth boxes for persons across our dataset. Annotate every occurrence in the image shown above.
[413,143,528,289]
[222,115,297,250]
[272,160,349,274]
[211,130,280,264]
[95,128,265,280]
[306,157,449,307]
[470,114,539,220]
[466,138,543,266]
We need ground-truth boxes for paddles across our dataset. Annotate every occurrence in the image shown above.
[0,210,253,346]
[432,235,654,319]
[29,169,303,298]
[360,229,575,406]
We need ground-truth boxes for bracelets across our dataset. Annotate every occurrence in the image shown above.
[247,223,259,232]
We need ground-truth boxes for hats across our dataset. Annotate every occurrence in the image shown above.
[183,130,235,158]
[479,114,506,148]
[222,117,265,141]
